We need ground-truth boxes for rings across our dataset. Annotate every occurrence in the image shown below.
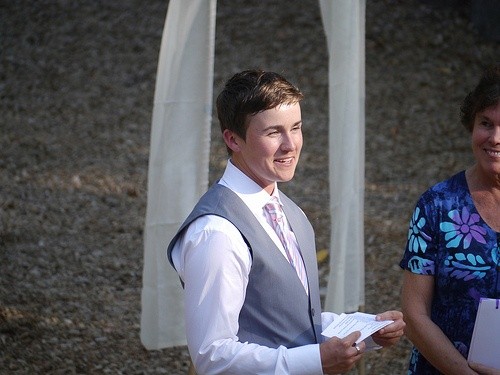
[355,346,360,356]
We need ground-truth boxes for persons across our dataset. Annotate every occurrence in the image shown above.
[398,68,500,375]
[167,71,407,375]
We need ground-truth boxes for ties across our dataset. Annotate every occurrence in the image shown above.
[264,203,309,295]
